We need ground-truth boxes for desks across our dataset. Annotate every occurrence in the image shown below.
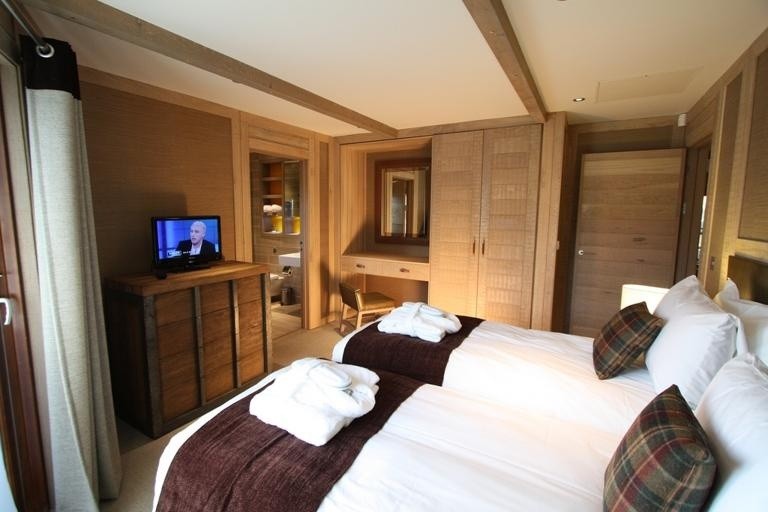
[103,260,273,440]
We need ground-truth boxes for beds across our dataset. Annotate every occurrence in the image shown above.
[149,357,635,512]
[331,303,658,436]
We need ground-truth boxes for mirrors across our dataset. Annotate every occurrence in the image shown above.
[374,157,431,246]
[280,160,300,236]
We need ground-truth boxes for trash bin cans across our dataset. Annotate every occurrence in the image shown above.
[281,287,294,305]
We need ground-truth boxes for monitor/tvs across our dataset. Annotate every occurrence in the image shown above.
[151,216,222,273]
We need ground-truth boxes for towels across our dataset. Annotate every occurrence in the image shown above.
[263,203,282,213]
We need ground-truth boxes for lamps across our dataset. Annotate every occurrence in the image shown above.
[620,283,670,315]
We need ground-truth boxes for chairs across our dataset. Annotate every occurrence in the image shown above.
[338,282,395,333]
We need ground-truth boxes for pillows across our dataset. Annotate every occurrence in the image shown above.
[593,301,664,379]
[693,353,768,512]
[644,275,737,410]
[713,277,768,368]
[603,384,717,512]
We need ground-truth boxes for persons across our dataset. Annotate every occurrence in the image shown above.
[173,220,216,255]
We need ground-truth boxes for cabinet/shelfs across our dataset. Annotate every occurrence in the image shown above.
[427,112,579,332]
[259,159,284,234]
[341,252,429,282]
